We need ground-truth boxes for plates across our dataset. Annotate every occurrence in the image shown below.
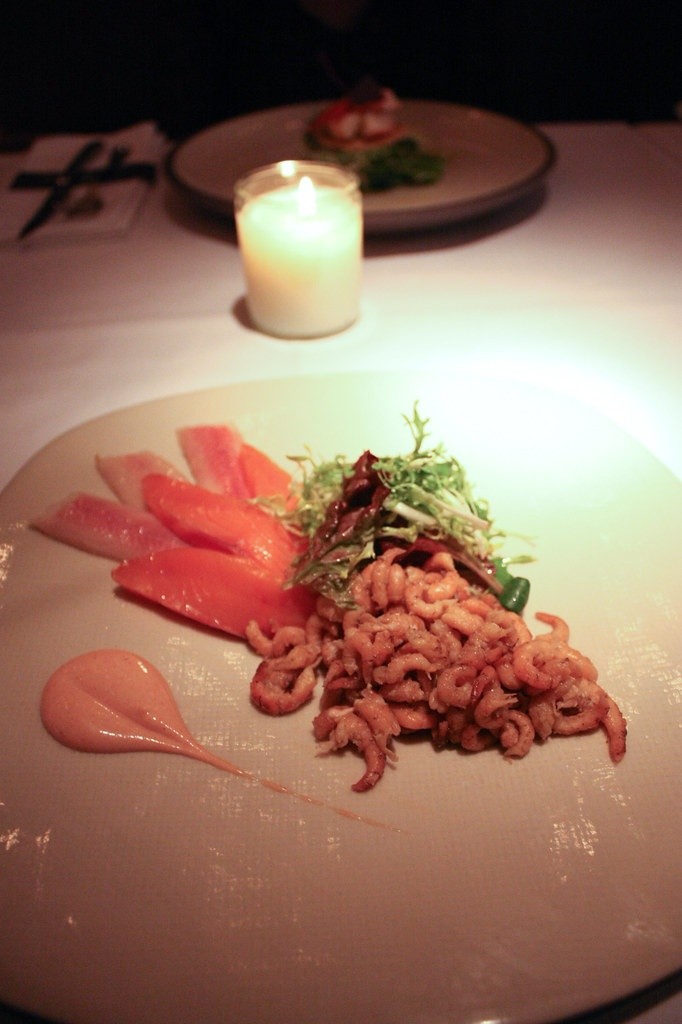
[165,99,555,238]
[0,376,682,1024]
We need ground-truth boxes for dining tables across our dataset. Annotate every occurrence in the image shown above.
[0,118,682,1024]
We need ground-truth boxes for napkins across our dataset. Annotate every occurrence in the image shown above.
[0,121,165,242]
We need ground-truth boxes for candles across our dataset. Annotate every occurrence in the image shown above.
[234,185,366,336]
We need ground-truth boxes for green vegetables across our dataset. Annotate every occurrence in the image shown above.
[299,135,445,192]
[247,399,535,614]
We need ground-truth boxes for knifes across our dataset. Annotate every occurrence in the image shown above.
[18,139,104,240]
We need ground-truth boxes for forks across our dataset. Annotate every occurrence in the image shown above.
[66,142,128,221]
